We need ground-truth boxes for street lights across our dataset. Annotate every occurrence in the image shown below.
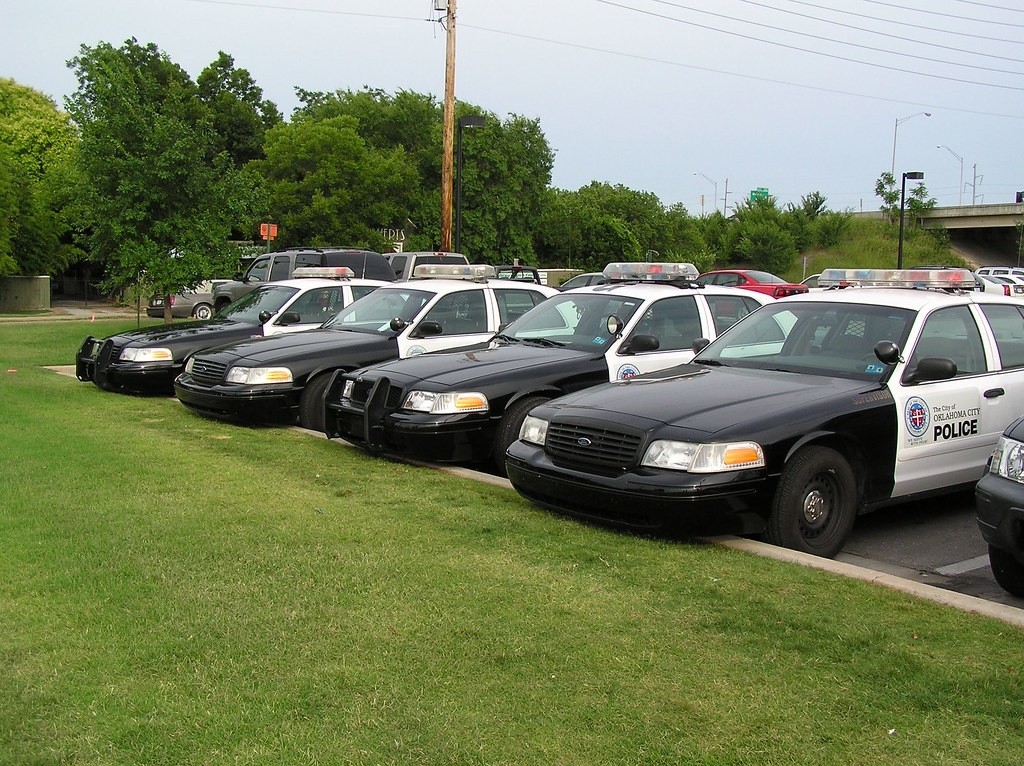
[896,171,924,270]
[694,171,717,212]
[454,115,486,254]
[888,111,931,209]
[937,144,964,207]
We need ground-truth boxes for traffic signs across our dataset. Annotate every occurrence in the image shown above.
[751,187,769,205]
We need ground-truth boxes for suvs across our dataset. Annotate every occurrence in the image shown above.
[209,246,398,317]
[380,251,473,284]
[491,264,542,286]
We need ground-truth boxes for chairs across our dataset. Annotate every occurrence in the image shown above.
[320,301,341,323]
[820,335,867,364]
[303,302,322,323]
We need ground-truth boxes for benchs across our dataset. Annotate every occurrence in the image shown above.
[913,336,1023,374]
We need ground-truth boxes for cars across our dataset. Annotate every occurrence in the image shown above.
[171,263,614,433]
[796,263,1024,338]
[323,262,801,476]
[974,416,1024,599]
[72,265,425,399]
[501,268,1023,561]
[147,290,214,321]
[691,269,812,301]
[557,271,639,293]
